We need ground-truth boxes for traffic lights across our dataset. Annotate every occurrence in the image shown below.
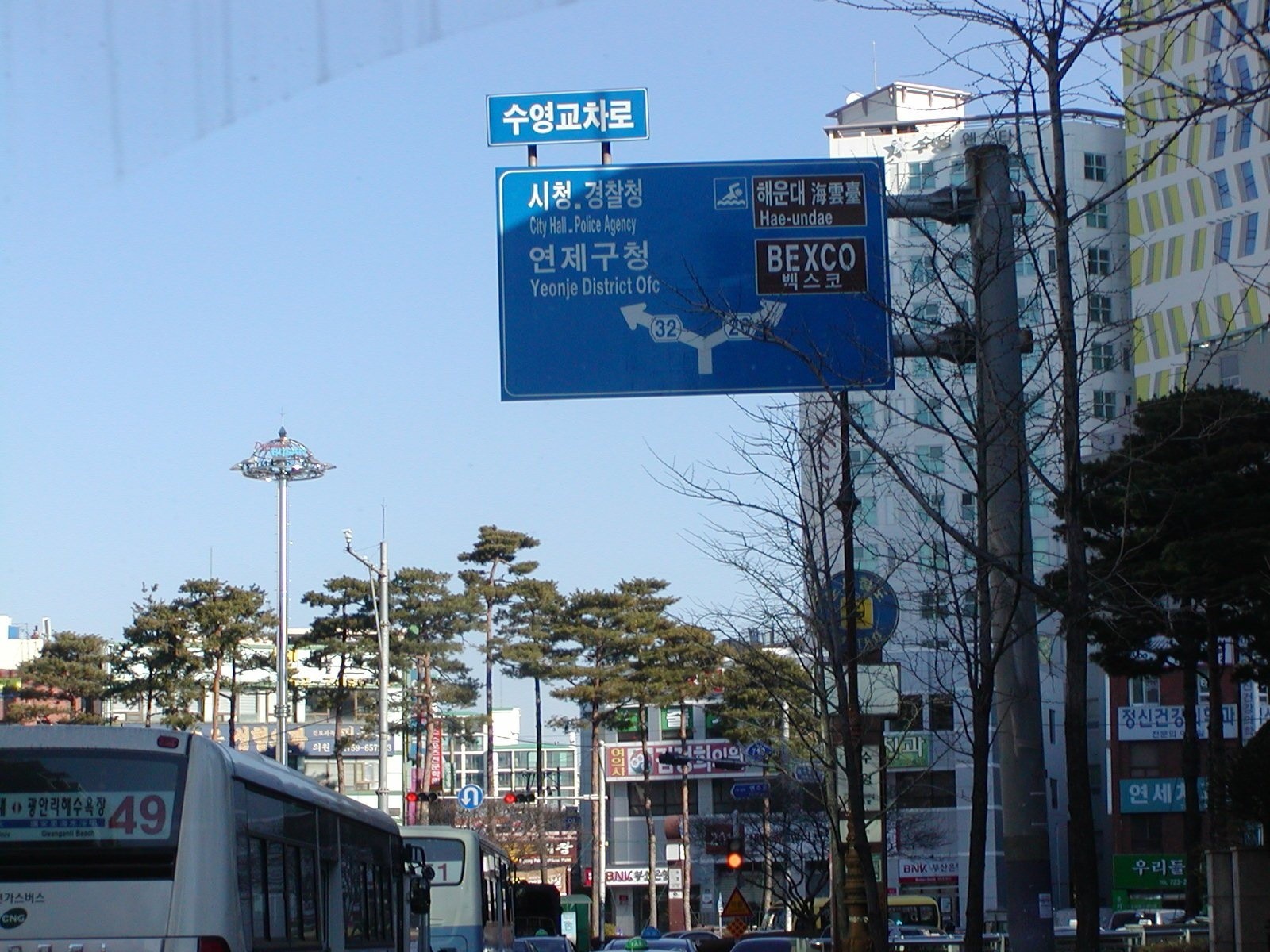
[727,838,742,868]
[407,791,438,802]
[504,792,535,802]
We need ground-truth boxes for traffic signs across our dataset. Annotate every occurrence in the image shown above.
[494,158,896,402]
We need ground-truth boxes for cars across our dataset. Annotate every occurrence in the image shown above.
[731,923,949,952]
[603,926,695,952]
[661,930,721,946]
[516,936,575,952]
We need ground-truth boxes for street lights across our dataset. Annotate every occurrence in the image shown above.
[231,425,335,764]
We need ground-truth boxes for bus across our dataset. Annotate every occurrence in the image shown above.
[400,826,516,952]
[513,881,562,936]
[761,895,940,932]
[0,724,432,952]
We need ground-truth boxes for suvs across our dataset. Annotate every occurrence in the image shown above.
[1109,910,1184,932]
